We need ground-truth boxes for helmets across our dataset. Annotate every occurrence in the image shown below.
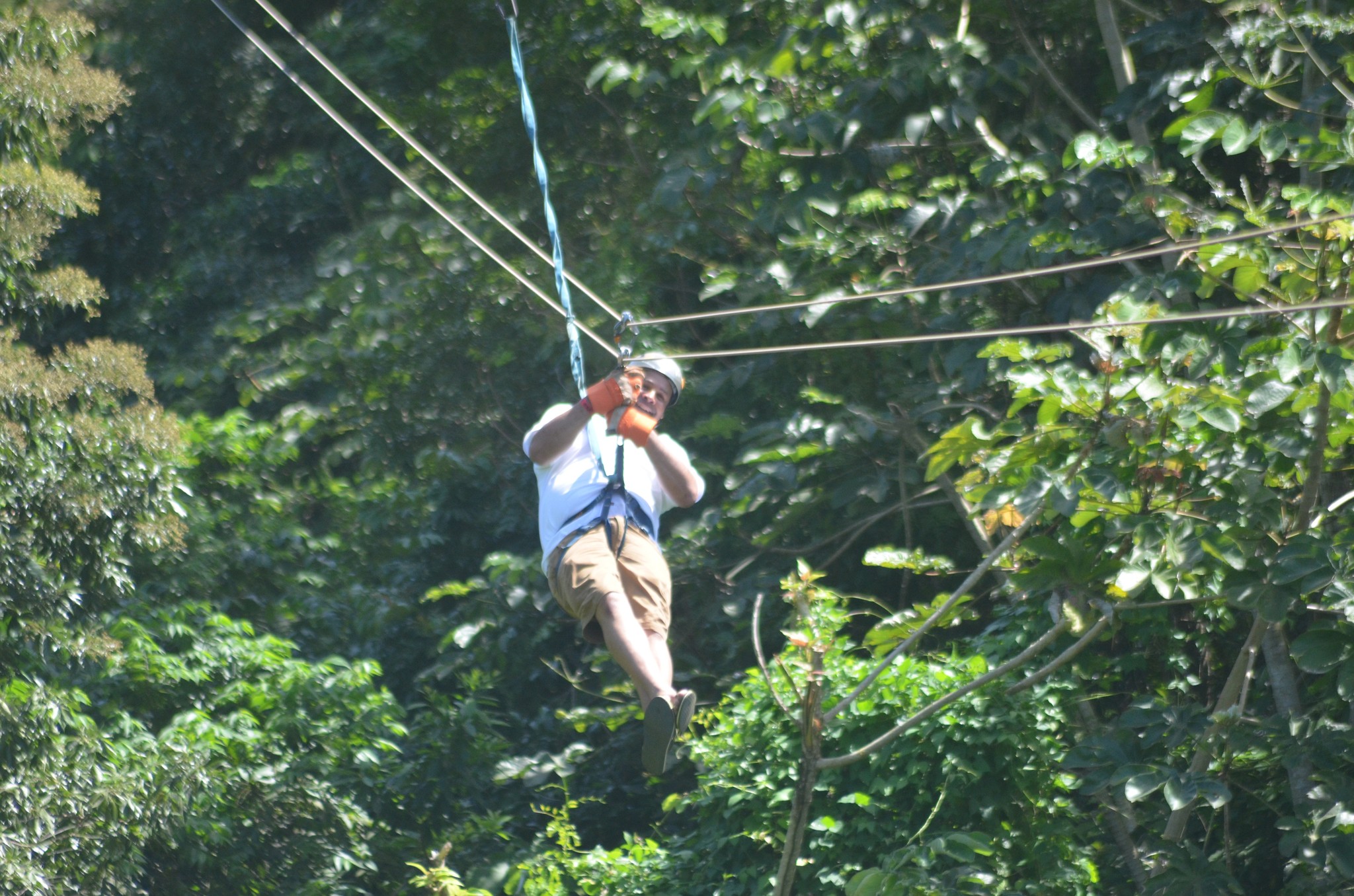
[628,348,685,407]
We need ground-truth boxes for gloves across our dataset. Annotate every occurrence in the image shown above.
[605,405,658,448]
[587,365,643,414]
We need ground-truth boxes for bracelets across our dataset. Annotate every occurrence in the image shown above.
[579,398,595,416]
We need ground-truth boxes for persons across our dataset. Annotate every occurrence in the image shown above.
[519,352,705,778]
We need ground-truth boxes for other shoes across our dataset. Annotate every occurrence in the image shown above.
[673,690,696,736]
[641,697,677,776]
